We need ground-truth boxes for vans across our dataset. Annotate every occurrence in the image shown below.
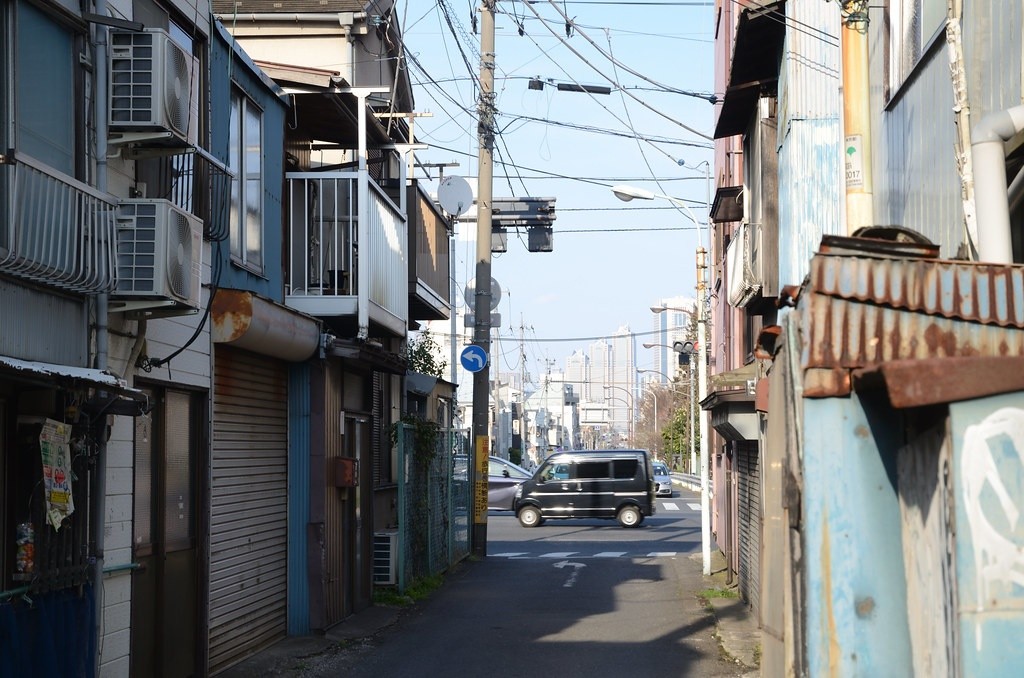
[512,448,658,528]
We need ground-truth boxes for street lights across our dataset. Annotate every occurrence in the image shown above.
[612,183,713,574]
[603,386,635,449]
[636,367,675,471]
[605,397,630,450]
[631,387,657,461]
[644,343,696,473]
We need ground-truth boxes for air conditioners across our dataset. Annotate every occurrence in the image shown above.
[103,22,201,157]
[106,197,207,320]
[372,524,401,585]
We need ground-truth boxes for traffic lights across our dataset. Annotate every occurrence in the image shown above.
[673,340,700,353]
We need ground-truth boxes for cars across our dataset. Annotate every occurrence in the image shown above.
[449,453,536,514]
[650,461,672,498]
